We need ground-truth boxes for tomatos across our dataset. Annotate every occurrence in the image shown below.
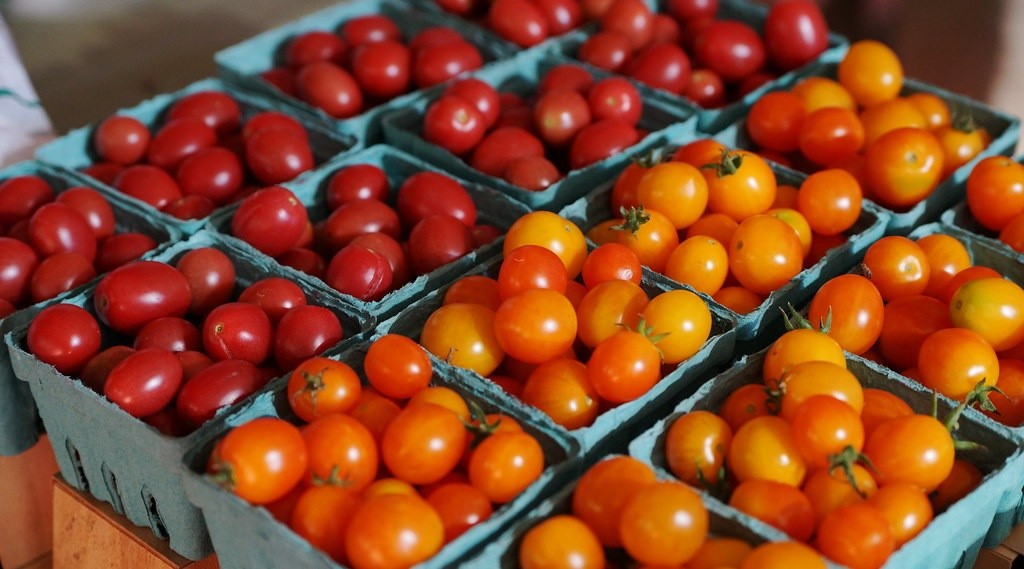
[0,0,1024,568]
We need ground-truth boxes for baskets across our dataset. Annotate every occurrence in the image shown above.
[0,0,1024,569]
